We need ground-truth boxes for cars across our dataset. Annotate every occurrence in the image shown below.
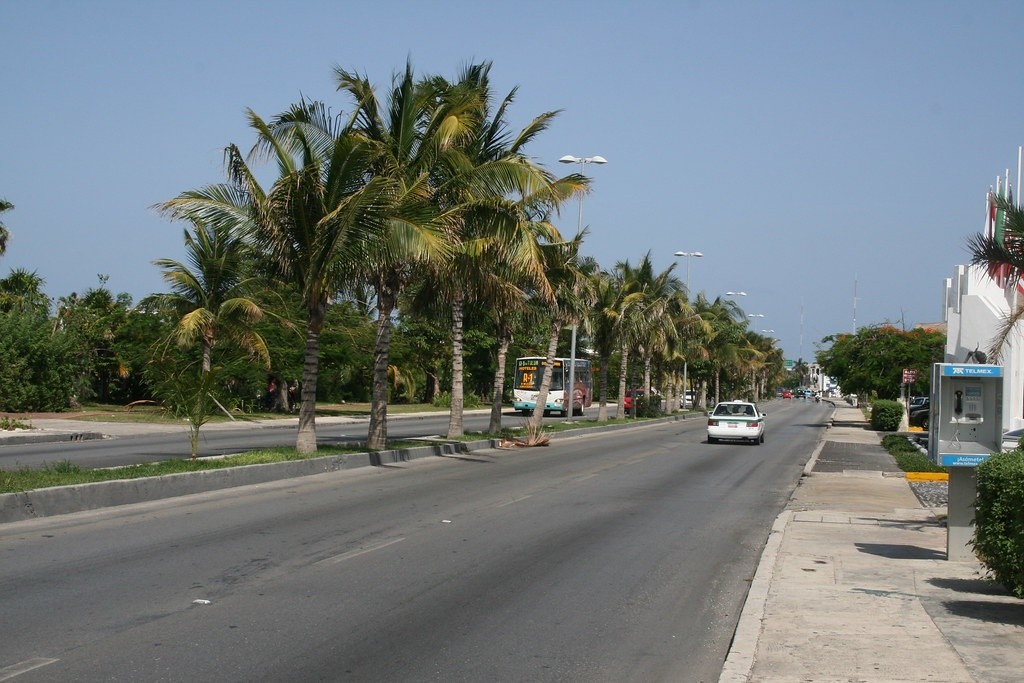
[623,389,656,414]
[796,388,815,399]
[776,392,783,397]
[909,396,930,429]
[782,391,792,400]
[707,400,767,446]
[679,390,701,409]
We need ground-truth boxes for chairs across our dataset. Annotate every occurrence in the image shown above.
[743,406,752,413]
[719,406,727,412]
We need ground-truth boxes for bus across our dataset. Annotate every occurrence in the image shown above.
[511,357,594,418]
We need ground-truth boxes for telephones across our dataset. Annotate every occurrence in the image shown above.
[949,383,985,425]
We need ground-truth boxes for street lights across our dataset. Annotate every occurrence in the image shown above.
[760,330,775,340]
[748,314,764,335]
[559,155,608,423]
[725,292,749,307]
[673,251,704,408]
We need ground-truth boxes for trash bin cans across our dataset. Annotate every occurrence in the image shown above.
[852,394,858,407]
